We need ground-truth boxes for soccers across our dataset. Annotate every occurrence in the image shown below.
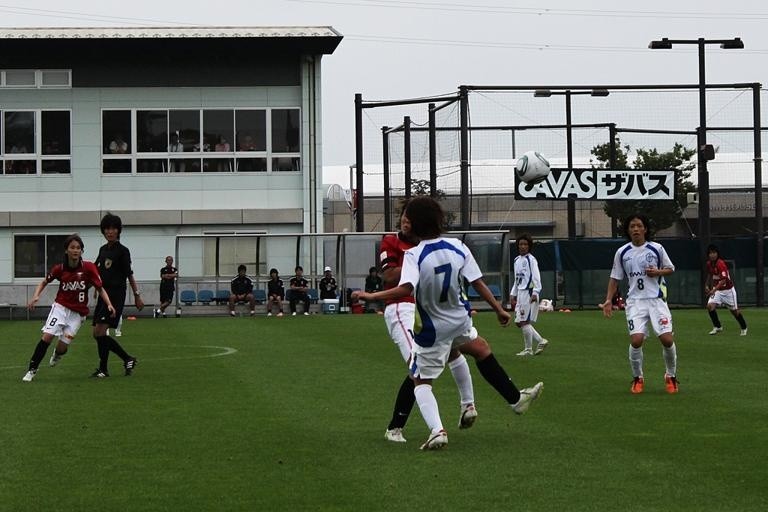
[515,151,550,184]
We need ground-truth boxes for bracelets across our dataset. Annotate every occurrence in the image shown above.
[134,291,140,296]
[713,287,716,290]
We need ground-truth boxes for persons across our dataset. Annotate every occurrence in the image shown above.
[378,199,544,444]
[509,234,547,357]
[599,213,680,395]
[153,256,178,319]
[351,195,510,450]
[9,139,27,174]
[22,234,117,382]
[705,244,748,337]
[90,212,144,379]
[104,130,257,172]
[228,265,383,317]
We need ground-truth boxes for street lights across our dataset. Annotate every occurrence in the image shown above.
[535,86,611,243]
[648,35,745,311]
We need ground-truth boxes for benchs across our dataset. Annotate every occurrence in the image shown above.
[467,283,503,298]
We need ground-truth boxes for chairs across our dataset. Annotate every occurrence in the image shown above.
[180,285,340,307]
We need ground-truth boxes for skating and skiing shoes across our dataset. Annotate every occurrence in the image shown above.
[510,381,543,414]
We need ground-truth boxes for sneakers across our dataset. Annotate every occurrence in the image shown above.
[23,371,36,381]
[89,370,109,378]
[153,308,168,318]
[516,350,533,356]
[124,356,136,376]
[664,373,679,394]
[50,349,61,367]
[420,428,448,449]
[708,326,723,334]
[230,311,311,316]
[459,404,477,428]
[535,339,548,354]
[631,376,644,393]
[384,427,406,442]
[740,328,747,336]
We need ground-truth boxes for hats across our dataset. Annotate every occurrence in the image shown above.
[324,267,332,271]
